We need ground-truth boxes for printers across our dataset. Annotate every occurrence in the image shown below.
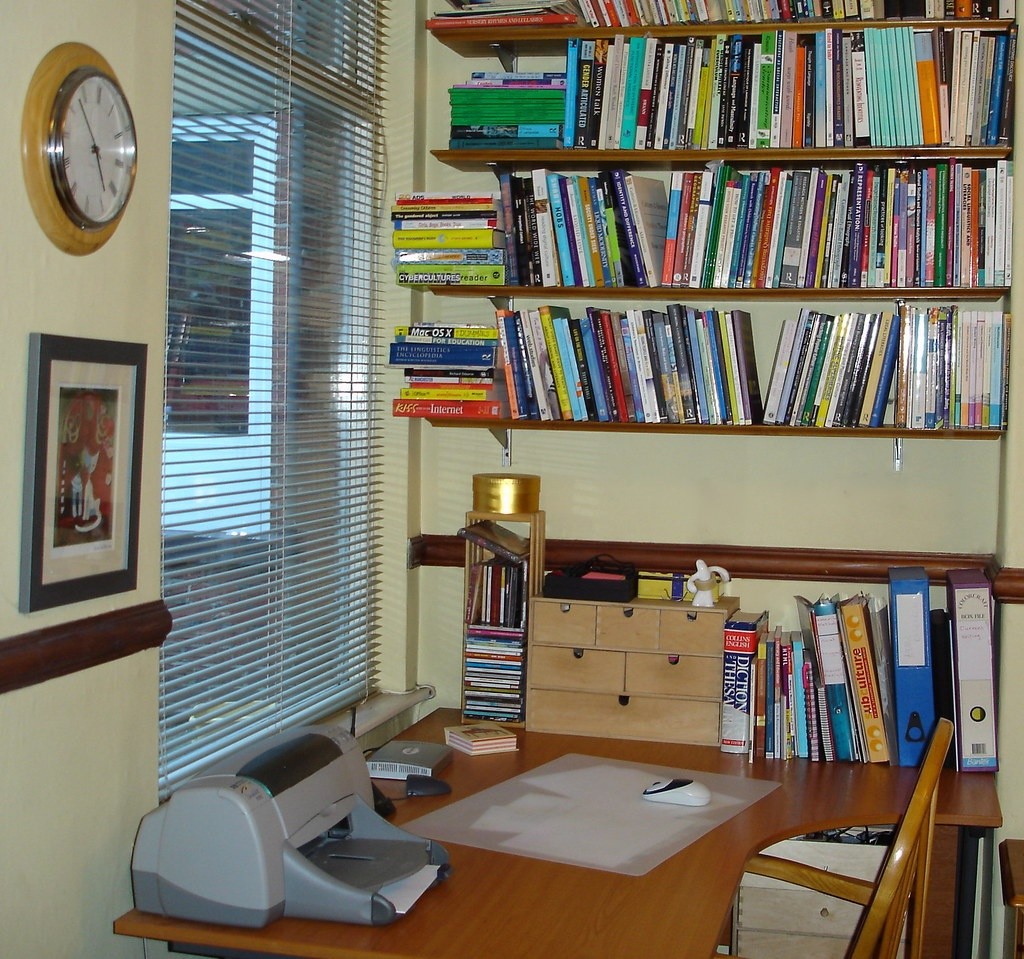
[132,725,454,929]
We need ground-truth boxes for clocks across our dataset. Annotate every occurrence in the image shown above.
[21,42,139,256]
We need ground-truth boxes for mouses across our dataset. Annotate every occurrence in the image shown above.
[642,778,711,807]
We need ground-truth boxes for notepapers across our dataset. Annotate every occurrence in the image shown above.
[443,721,519,757]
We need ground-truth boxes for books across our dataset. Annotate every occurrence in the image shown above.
[385,0,1017,770]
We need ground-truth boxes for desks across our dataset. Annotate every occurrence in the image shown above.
[113,709,1002,959]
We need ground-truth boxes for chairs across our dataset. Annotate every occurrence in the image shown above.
[714,718,955,959]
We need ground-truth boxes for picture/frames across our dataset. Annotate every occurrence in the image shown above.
[19,333,147,613]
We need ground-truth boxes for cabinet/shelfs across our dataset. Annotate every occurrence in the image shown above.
[426,19,1014,446]
[460,508,740,743]
